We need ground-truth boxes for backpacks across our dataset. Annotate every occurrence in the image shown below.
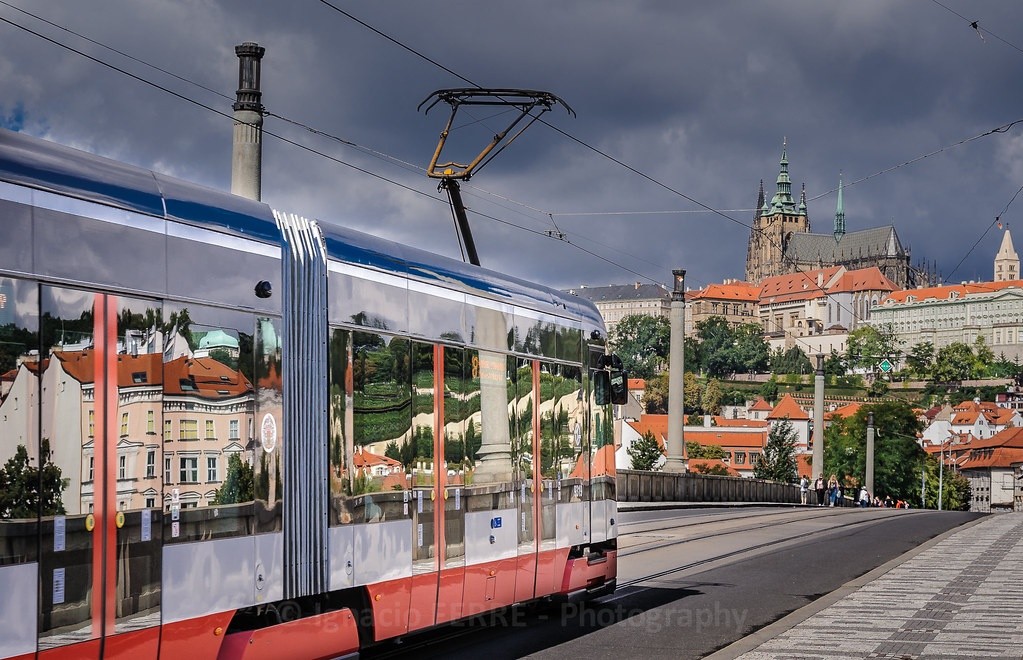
[803,478,808,488]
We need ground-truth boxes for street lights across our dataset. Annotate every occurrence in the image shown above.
[939,437,956,511]
[922,438,931,508]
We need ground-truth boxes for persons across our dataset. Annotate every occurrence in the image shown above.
[800,475,808,505]
[859,486,871,508]
[838,481,844,506]
[814,473,827,506]
[827,475,839,507]
[875,495,909,509]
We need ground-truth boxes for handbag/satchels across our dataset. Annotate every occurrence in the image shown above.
[836,490,841,498]
[828,489,832,495]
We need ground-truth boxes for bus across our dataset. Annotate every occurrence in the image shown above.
[0,130,630,660]
